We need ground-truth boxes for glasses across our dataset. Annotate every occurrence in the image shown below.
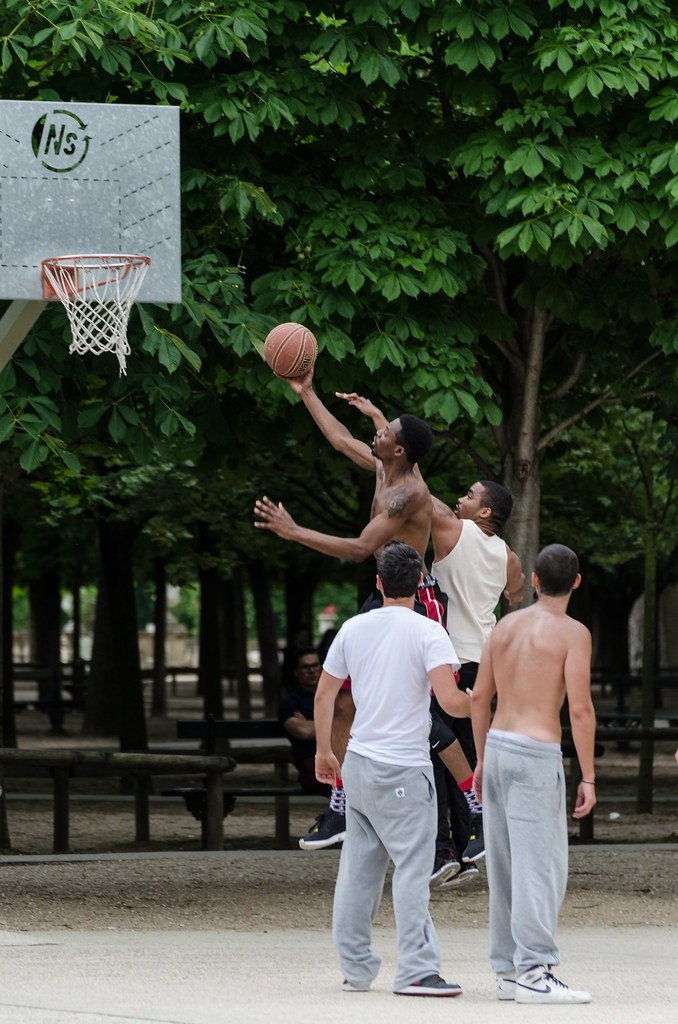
[298,662,321,672]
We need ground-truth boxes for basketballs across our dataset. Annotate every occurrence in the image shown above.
[264,322,318,378]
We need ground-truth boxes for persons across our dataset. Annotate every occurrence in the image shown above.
[335,392,527,891]
[251,323,489,861]
[282,646,335,796]
[472,546,597,1007]
[314,540,472,995]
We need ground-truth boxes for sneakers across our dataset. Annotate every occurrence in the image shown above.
[517,963,591,1004]
[342,978,368,991]
[496,968,517,1000]
[462,812,485,862]
[429,847,460,889]
[299,808,347,850]
[432,850,479,891]
[393,974,463,997]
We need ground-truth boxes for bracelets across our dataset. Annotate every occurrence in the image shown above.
[581,779,596,784]
